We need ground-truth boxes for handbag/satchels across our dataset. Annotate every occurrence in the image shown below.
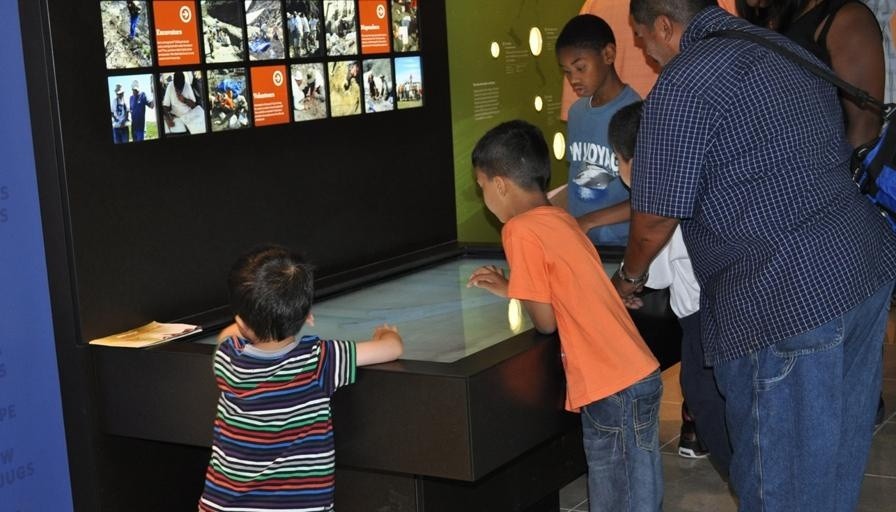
[848,104,896,231]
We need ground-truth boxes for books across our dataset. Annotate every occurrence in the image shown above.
[88,320,203,348]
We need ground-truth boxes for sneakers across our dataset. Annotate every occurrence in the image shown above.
[678,401,710,458]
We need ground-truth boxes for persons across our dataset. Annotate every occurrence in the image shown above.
[212,87,249,126]
[286,11,320,57]
[368,73,389,101]
[397,81,422,100]
[252,21,280,41]
[126,0,141,39]
[291,71,325,112]
[196,243,405,512]
[110,80,155,144]
[207,20,231,59]
[162,72,197,127]
